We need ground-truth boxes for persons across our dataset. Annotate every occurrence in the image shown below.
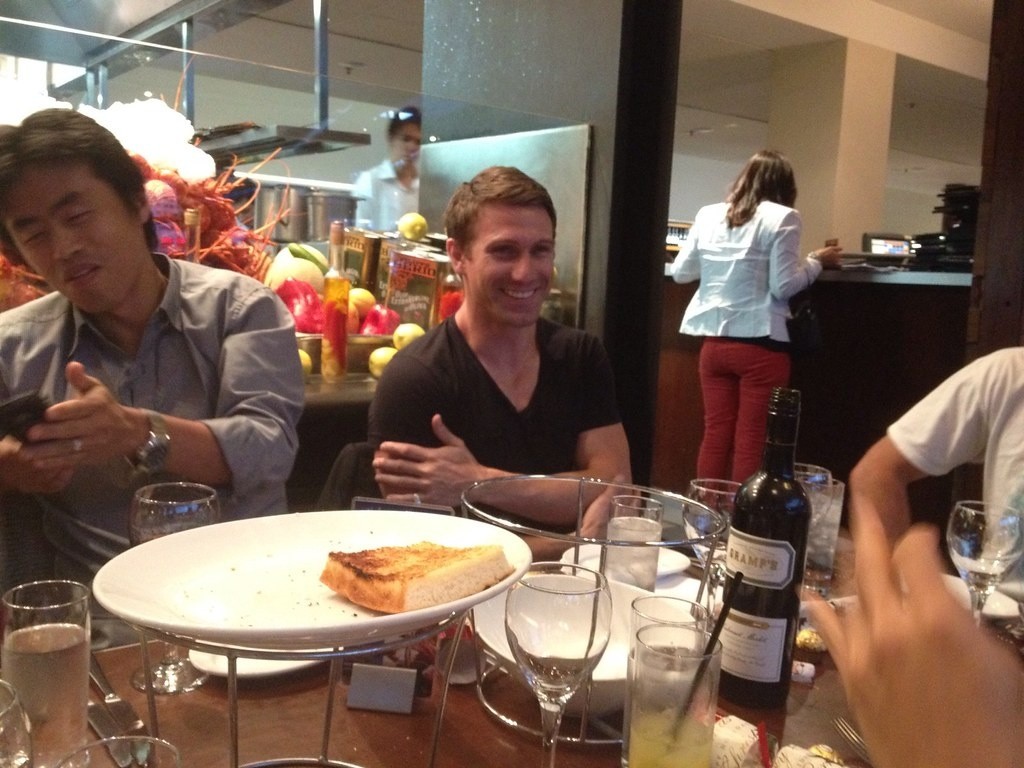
[670,150,841,515]
[366,167,641,563]
[851,346,1024,637]
[795,495,1024,768]
[0,110,305,653]
[353,106,421,233]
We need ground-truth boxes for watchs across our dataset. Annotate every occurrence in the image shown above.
[125,408,171,479]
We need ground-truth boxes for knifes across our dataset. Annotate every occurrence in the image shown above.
[83,652,152,768]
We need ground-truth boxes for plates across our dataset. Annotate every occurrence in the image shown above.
[188,637,344,678]
[562,544,724,613]
[91,509,533,652]
[940,572,1021,625]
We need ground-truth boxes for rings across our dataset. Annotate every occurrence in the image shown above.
[72,437,82,453]
[413,492,420,504]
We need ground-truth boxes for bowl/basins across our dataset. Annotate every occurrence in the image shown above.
[467,575,705,720]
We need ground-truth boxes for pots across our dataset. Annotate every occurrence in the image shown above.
[258,185,366,246]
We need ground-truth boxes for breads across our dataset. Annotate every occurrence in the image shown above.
[320,541,514,613]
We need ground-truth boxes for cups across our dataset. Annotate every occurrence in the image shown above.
[600,495,665,594]
[54,736,183,768]
[0,678,35,768]
[1,579,92,768]
[794,461,845,596]
[622,595,722,768]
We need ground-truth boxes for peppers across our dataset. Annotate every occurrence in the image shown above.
[277,277,401,336]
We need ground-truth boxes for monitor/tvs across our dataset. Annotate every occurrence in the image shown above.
[862,232,912,255]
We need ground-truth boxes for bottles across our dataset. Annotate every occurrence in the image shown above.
[182,207,201,266]
[321,221,351,383]
[711,386,812,711]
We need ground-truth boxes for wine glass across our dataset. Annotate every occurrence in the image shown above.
[129,481,220,698]
[506,562,614,768]
[681,478,742,635]
[947,500,1024,628]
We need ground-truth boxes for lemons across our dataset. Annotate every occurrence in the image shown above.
[298,288,426,378]
[396,212,427,241]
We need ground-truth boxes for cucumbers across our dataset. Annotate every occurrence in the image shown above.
[288,241,329,275]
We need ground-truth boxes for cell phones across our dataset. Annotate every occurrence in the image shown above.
[0,390,56,449]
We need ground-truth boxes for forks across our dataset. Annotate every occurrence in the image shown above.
[830,716,871,766]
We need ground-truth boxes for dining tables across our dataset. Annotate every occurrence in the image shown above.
[0,531,1024,768]
